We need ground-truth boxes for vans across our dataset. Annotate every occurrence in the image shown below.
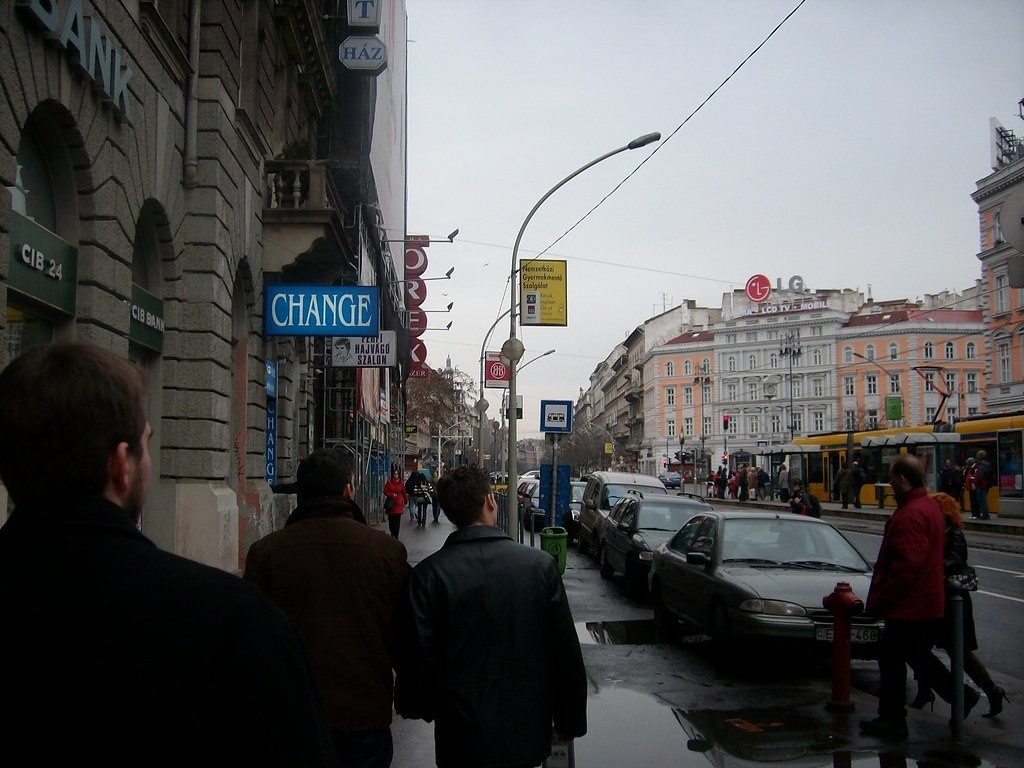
[577,470,669,560]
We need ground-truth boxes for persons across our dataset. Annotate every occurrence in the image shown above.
[940,450,996,521]
[857,454,980,740]
[404,465,451,528]
[401,458,587,768]
[908,492,1010,718]
[2,341,340,768]
[833,461,864,508]
[383,469,408,541]
[706,464,789,502]
[241,445,432,768]
[789,478,812,515]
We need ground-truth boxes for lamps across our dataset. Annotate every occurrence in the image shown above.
[388,267,455,286]
[379,229,459,246]
[396,302,454,315]
[405,321,452,332]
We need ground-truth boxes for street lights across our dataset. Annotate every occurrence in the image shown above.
[778,347,804,441]
[496,348,558,533]
[504,130,661,534]
[852,352,905,427]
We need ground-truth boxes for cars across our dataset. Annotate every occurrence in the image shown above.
[490,468,594,544]
[646,507,890,675]
[658,471,685,489]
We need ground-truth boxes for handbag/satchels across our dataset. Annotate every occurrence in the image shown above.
[384,498,395,510]
[946,565,978,592]
[424,491,433,505]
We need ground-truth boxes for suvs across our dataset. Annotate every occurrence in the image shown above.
[597,490,718,599]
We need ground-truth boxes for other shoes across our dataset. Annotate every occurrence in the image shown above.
[969,515,979,519]
[860,718,909,742]
[433,517,440,524]
[949,688,981,726]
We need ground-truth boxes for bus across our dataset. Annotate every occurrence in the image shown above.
[787,363,1024,518]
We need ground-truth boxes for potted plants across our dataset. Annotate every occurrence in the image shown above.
[273,137,314,208]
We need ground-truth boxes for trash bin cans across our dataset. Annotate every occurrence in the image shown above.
[707,481,714,498]
[539,525,568,576]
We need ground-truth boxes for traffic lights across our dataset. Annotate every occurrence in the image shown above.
[721,455,728,464]
[674,453,678,460]
[724,416,728,430]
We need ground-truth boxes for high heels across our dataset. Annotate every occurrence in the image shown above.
[911,691,935,711]
[982,686,1011,718]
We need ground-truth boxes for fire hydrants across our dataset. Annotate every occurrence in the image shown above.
[822,580,864,716]
